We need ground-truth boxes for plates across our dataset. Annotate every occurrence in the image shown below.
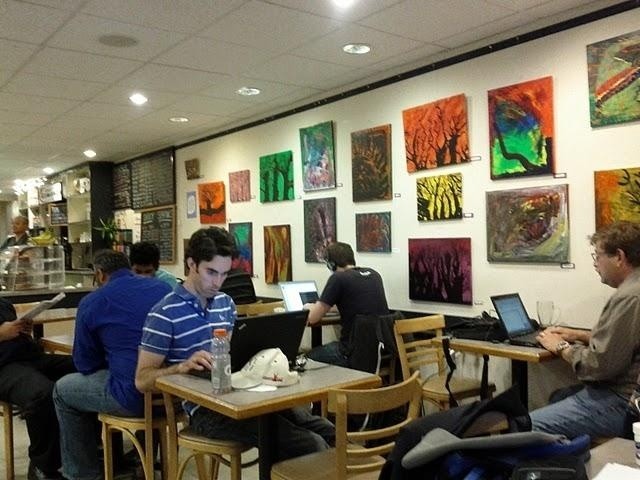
[30,161,113,272]
[1,246,66,291]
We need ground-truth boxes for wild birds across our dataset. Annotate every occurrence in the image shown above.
[278,280,337,318]
[490,293,543,348]
[189,308,311,382]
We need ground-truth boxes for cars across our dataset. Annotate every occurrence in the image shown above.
[633,423,640,468]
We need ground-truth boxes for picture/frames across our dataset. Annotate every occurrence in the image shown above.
[38,333,74,355]
[154,358,382,480]
[431,332,574,416]
[306,312,340,351]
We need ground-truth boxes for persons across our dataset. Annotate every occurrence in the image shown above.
[0,289,73,480]
[529,219,640,437]
[308,241,388,372]
[52,249,172,480]
[131,242,177,289]
[134,226,335,460]
[0,216,30,250]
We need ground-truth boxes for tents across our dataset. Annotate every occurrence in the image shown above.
[325,244,337,270]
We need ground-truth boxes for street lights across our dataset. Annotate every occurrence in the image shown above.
[439,311,541,408]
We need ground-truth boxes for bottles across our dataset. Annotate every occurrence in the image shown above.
[231,347,300,390]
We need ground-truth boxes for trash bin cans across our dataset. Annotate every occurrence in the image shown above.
[556,341,568,353]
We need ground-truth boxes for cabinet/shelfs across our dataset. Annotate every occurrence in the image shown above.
[210,328,232,396]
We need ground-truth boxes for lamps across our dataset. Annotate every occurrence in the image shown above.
[394,313,496,419]
[271,370,423,480]
[236,302,284,316]
[0,403,23,480]
[176,402,259,480]
[98,392,206,480]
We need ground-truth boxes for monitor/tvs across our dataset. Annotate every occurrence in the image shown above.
[19,290,65,322]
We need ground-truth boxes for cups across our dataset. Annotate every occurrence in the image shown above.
[591,252,605,262]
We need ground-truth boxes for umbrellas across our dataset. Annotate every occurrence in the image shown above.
[27,462,68,480]
